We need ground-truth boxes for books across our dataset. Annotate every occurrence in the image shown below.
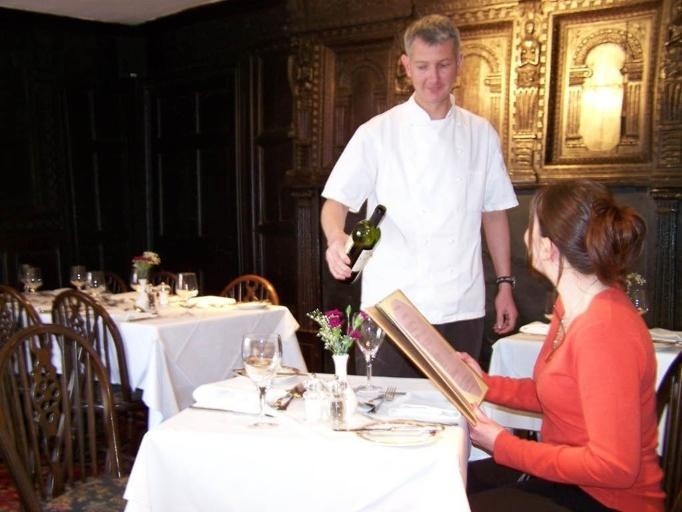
[369,289,491,424]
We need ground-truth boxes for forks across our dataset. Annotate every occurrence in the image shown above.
[385,385,397,402]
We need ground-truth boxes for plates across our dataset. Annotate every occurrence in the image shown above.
[652,339,676,349]
[518,323,548,336]
[234,365,301,381]
[235,302,267,309]
[357,419,439,445]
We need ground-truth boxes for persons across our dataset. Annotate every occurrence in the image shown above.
[455,177,668,512]
[319,14,520,378]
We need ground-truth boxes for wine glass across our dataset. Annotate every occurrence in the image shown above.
[176,272,199,308]
[18,264,44,298]
[351,313,384,395]
[70,265,86,290]
[240,332,282,428]
[85,271,106,303]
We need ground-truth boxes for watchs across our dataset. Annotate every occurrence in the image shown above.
[496,276,517,289]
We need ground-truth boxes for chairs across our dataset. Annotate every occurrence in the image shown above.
[525,347,681,511]
[53,290,149,472]
[1,290,130,512]
[222,276,280,307]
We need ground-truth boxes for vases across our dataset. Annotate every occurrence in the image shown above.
[626,288,648,313]
[331,351,350,382]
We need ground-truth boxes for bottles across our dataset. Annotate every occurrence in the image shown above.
[136,282,171,313]
[331,204,387,286]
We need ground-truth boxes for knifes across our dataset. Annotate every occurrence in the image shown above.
[332,424,446,433]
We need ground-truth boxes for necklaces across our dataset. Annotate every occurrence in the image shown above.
[551,279,597,350]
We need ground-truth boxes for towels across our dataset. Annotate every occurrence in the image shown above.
[648,324,681,344]
[360,386,469,433]
[516,320,548,335]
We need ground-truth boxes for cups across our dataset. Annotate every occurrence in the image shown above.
[545,291,556,320]
[306,372,348,431]
[130,268,139,291]
[628,287,647,316]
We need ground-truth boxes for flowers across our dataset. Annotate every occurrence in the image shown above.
[130,249,161,279]
[624,271,646,302]
[304,304,368,356]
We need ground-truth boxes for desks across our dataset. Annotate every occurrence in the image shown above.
[21,287,303,430]
